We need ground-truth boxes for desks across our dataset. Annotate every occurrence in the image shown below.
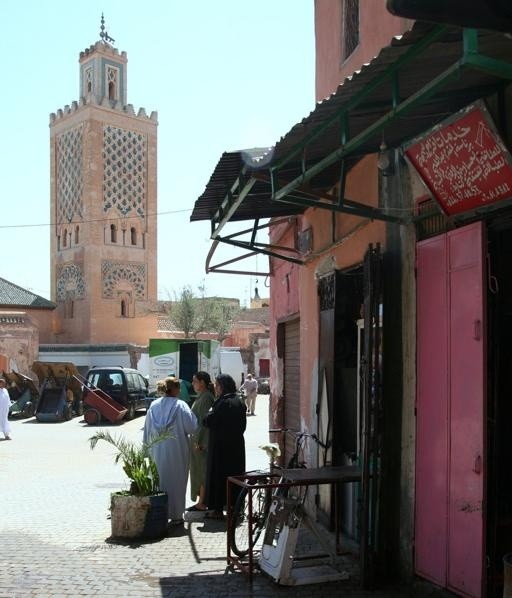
[226,465,376,590]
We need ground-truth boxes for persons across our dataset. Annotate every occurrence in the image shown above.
[238,373,258,416]
[168,372,192,405]
[201,375,247,519]
[184,371,216,512]
[0,378,13,440]
[143,376,198,525]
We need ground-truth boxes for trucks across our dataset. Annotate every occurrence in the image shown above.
[147,338,222,412]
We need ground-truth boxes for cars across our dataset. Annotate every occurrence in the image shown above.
[254,376,270,394]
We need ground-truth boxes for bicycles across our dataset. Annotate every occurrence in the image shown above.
[229,426,329,557]
[239,389,251,414]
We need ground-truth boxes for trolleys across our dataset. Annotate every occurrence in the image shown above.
[73,374,129,425]
[7,384,34,418]
[32,372,66,423]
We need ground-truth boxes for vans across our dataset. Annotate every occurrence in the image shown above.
[81,365,150,420]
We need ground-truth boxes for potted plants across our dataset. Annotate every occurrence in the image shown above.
[90,427,184,540]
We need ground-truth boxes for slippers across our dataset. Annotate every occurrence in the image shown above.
[203,511,223,519]
[187,506,206,511]
